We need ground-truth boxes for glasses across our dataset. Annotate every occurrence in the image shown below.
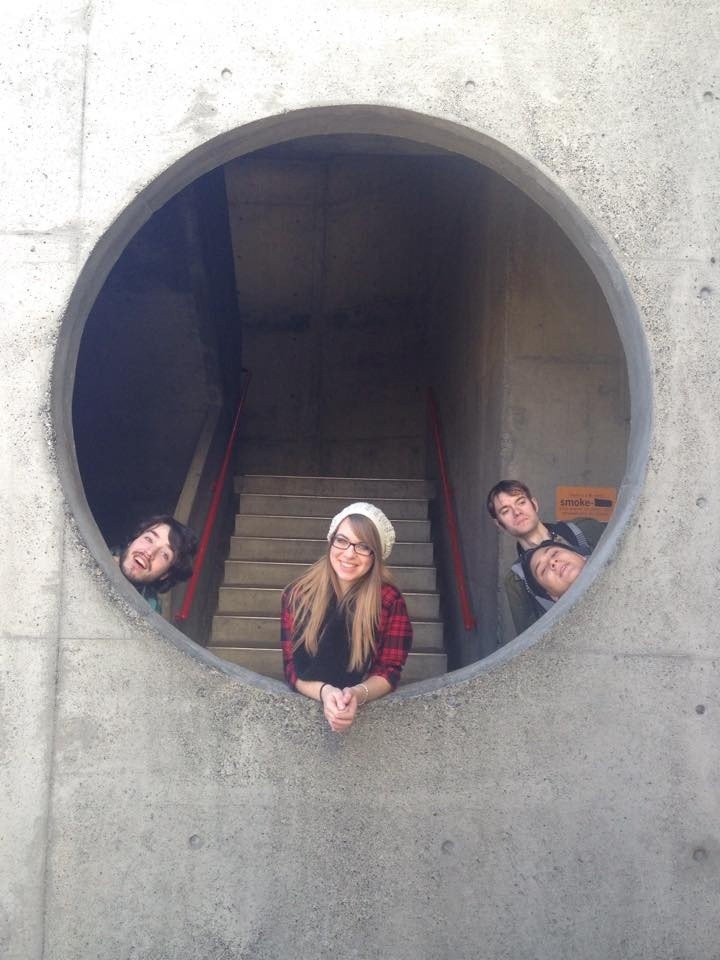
[331,537,373,556]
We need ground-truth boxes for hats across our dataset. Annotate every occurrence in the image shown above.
[328,502,395,560]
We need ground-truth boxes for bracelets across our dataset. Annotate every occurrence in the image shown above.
[355,684,369,706]
[320,683,330,704]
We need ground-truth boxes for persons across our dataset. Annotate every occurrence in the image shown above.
[280,501,414,733]
[520,540,591,605]
[104,516,200,616]
[487,479,607,637]
